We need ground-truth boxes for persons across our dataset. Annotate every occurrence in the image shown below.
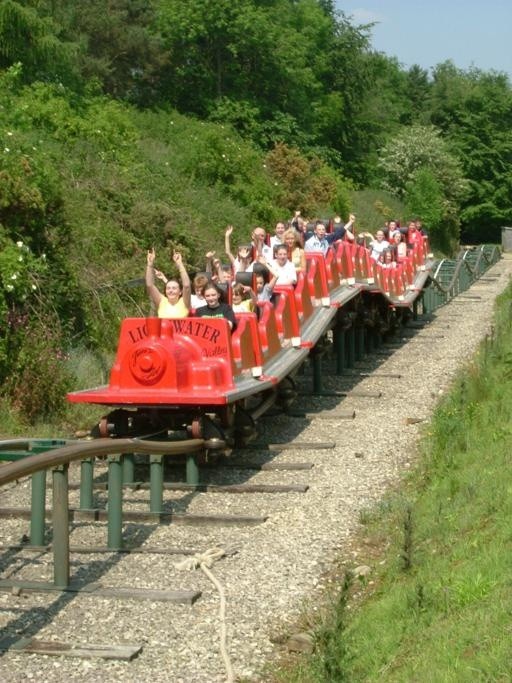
[153,267,209,309]
[145,246,192,317]
[194,279,238,333]
[204,204,429,314]
